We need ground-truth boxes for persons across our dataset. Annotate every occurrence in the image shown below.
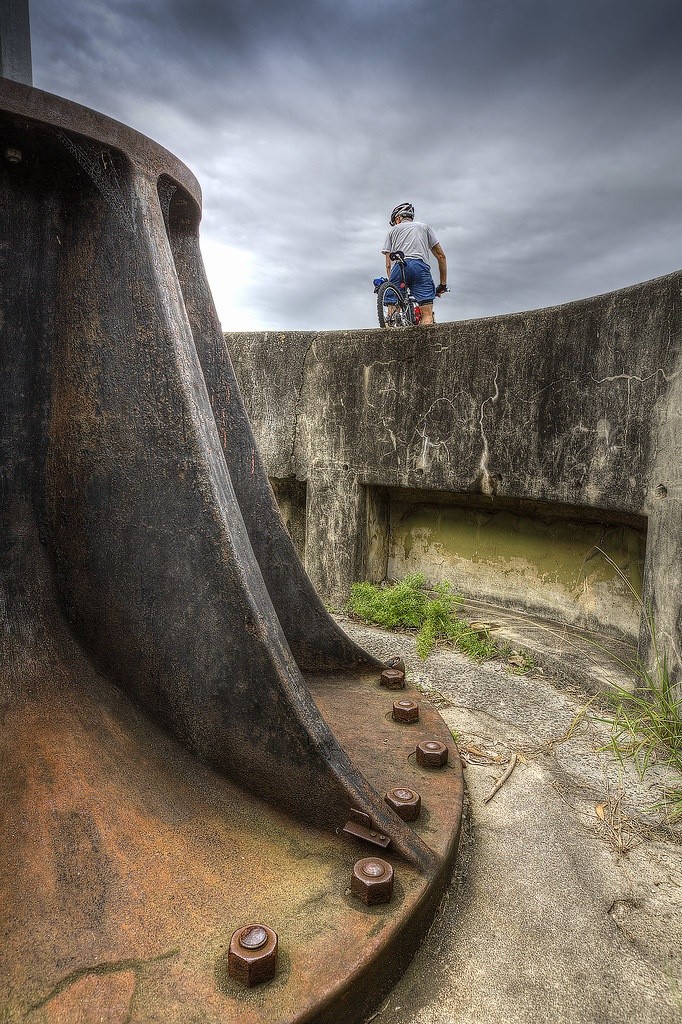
[381,203,447,326]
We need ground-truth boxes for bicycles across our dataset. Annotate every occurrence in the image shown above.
[372,250,451,328]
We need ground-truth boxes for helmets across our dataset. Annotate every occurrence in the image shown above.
[391,203,414,222]
